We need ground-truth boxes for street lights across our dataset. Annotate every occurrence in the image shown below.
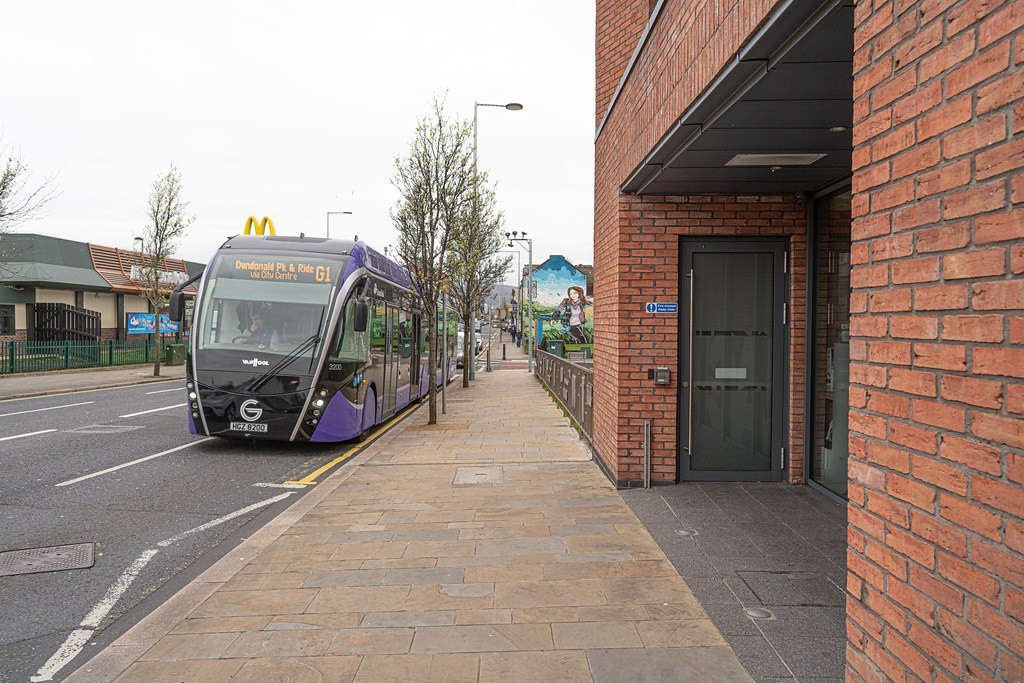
[470,100,524,381]
[134,237,143,255]
[327,212,352,238]
[505,231,532,371]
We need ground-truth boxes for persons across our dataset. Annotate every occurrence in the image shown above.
[237,308,279,348]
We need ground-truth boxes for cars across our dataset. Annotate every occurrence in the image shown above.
[456,319,509,367]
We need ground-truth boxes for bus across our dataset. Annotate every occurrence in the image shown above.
[169,232,459,443]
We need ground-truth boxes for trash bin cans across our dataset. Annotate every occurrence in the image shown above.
[546,339,564,359]
